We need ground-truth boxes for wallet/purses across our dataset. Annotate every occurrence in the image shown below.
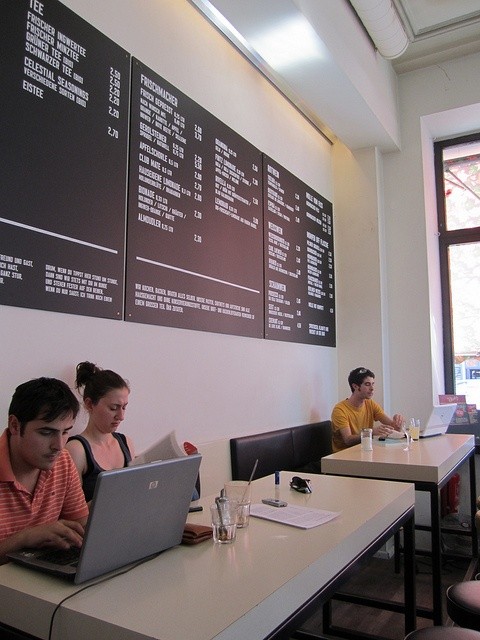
[183,522,212,545]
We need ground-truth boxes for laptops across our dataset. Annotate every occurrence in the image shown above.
[6,453,202,584]
[419,402,457,437]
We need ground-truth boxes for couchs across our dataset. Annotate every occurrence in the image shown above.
[229,420,335,480]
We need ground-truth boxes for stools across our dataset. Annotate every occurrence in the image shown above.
[404,626,480,640]
[445,581,480,628]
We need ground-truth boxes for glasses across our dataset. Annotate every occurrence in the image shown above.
[355,369,369,373]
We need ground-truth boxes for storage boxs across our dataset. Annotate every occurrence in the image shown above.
[440,513,473,557]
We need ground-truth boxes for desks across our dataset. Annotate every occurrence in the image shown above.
[321,434,479,626]
[0,470,416,639]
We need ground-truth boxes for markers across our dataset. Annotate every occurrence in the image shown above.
[275,471,279,503]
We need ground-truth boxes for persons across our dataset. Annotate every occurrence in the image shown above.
[331,368,404,454]
[0,376,90,557]
[60,361,150,519]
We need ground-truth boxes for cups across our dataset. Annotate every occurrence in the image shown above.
[225,481,252,528]
[210,502,237,544]
[361,428,373,450]
[413,419,420,441]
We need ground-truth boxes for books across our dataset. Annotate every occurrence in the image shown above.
[127,430,199,465]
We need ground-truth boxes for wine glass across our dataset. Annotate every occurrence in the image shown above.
[401,417,414,452]
